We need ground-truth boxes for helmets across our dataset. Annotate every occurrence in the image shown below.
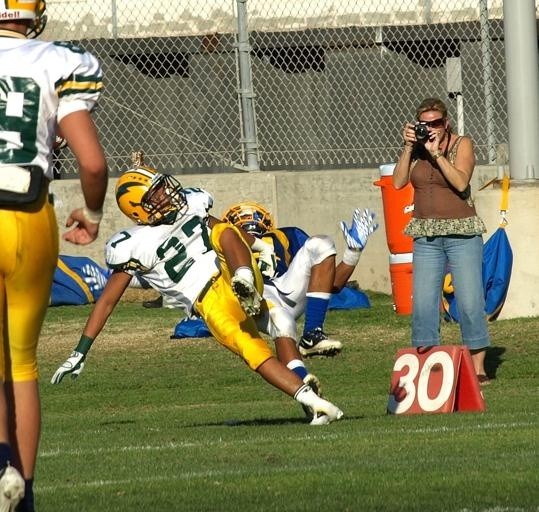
[0,0,46,23]
[224,204,272,234]
[114,165,184,226]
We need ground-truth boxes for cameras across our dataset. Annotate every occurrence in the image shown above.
[409,121,430,141]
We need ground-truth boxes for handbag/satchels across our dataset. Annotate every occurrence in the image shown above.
[439,226,513,324]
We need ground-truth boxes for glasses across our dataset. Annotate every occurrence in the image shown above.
[418,118,449,128]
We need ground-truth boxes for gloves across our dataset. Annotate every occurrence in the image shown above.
[338,207,380,251]
[49,347,89,387]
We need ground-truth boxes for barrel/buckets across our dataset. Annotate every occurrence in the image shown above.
[389,253,445,315]
[374,162,415,254]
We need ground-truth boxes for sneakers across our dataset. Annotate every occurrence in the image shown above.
[228,275,264,321]
[304,401,344,426]
[297,327,343,357]
[300,373,322,416]
[0,464,27,512]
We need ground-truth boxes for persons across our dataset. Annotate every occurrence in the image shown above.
[49,162,345,427]
[197,200,380,418]
[0,0,111,510]
[390,96,492,389]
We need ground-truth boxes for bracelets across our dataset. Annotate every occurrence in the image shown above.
[250,235,270,252]
[81,205,104,225]
[74,334,94,355]
[432,150,443,161]
[341,247,361,266]
